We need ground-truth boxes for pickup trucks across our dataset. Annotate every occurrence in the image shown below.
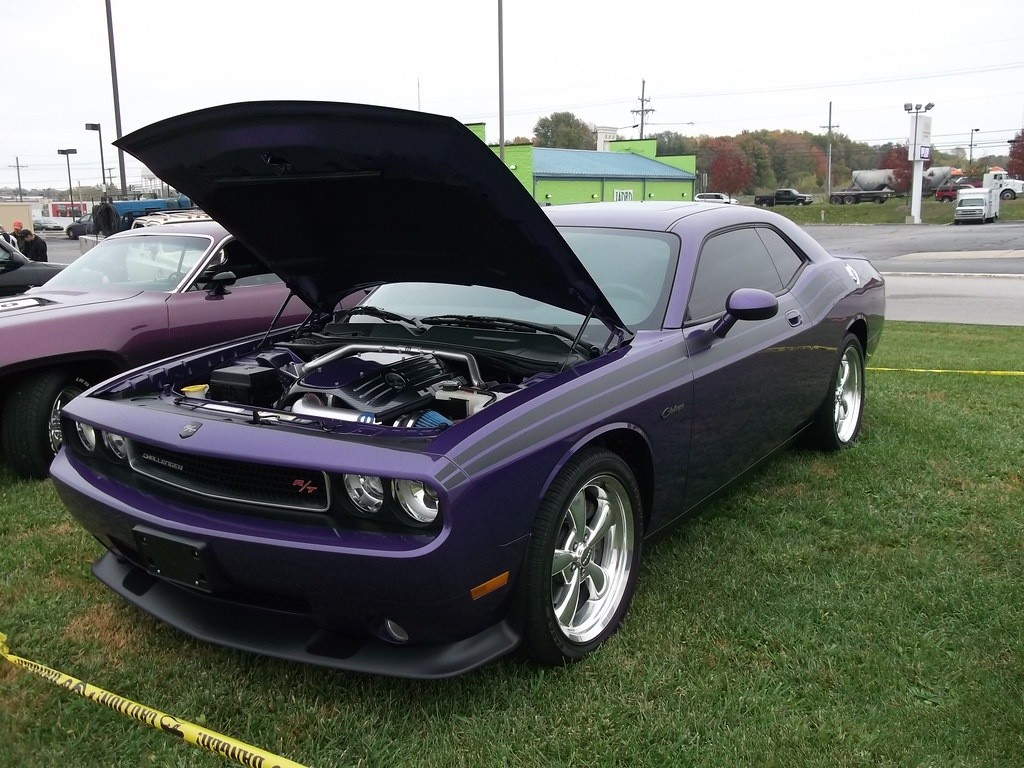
[753,188,814,207]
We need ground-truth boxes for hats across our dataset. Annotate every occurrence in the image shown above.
[13,219,23,230]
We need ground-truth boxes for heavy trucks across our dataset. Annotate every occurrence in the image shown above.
[830,165,1023,206]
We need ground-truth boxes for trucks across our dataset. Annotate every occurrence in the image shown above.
[954,187,999,226]
[107,198,183,221]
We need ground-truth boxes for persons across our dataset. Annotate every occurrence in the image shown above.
[10,221,48,263]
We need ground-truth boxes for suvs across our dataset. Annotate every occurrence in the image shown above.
[692,192,738,204]
[935,185,969,203]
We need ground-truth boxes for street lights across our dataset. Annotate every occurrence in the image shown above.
[57,148,86,227]
[904,100,935,223]
[85,122,112,208]
[970,128,980,168]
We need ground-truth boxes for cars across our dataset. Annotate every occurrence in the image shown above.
[64,213,100,240]
[0,232,109,306]
[952,176,980,184]
[49,100,889,674]
[0,213,323,480]
[32,219,64,231]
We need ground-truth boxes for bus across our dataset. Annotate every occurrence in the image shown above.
[41,202,88,218]
